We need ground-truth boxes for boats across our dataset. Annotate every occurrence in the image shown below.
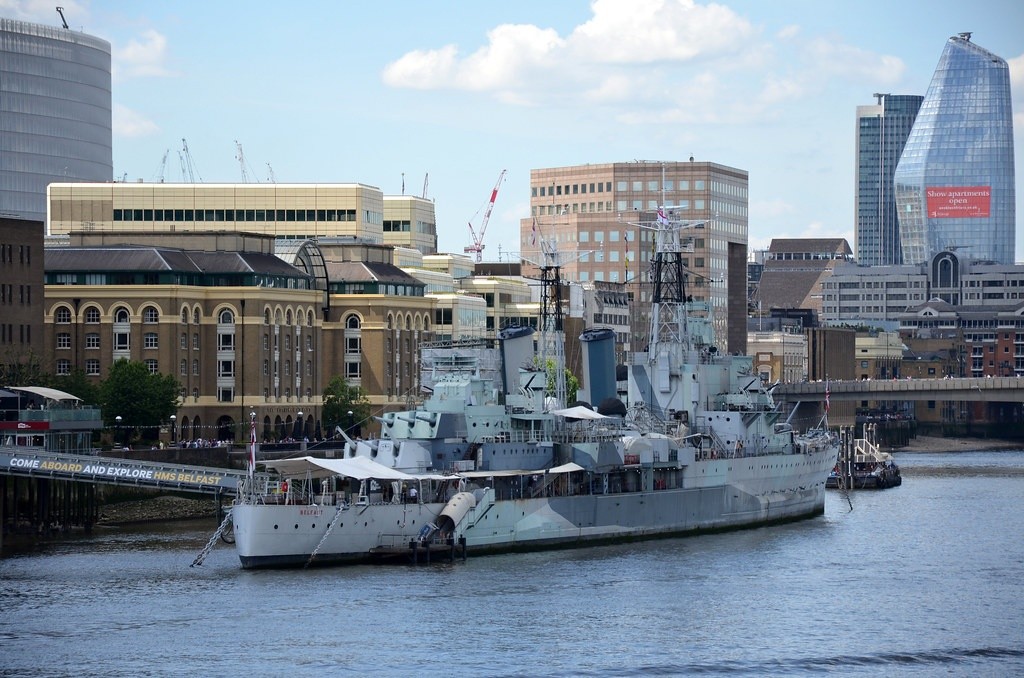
[221,164,841,572]
[825,420,904,491]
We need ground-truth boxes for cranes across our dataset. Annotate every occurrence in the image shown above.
[121,138,277,185]
[465,167,508,264]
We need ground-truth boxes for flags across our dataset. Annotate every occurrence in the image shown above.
[625,242,628,252]
[626,259,629,270]
[532,219,536,245]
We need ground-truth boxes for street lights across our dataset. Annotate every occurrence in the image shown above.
[169,414,178,445]
[250,412,256,441]
[116,415,122,451]
[348,410,354,438]
[296,411,304,440]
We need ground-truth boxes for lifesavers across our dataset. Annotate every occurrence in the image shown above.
[282,482,288,492]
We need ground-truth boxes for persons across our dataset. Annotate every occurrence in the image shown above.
[701,348,707,363]
[527,475,538,487]
[687,294,693,303]
[152,437,218,450]
[410,486,418,502]
[736,440,743,458]
